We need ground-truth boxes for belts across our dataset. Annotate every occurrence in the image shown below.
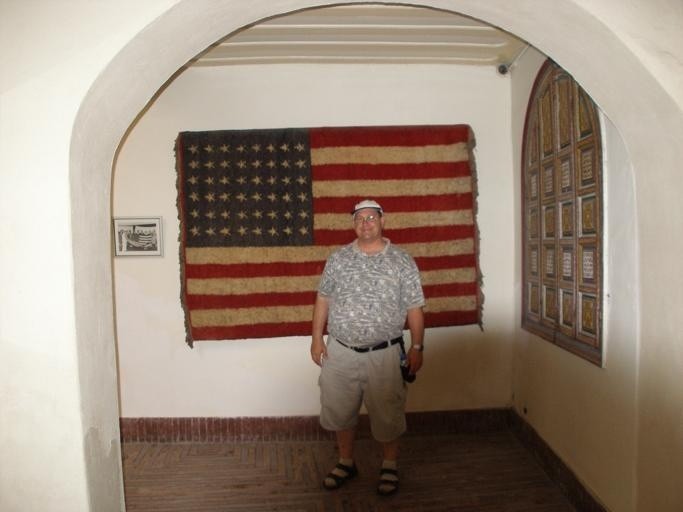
[335,336,402,353]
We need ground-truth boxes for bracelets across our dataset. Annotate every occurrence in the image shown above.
[409,343,424,352]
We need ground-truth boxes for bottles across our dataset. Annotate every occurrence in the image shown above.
[400,353,417,383]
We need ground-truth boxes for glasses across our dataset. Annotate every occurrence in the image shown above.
[353,214,380,224]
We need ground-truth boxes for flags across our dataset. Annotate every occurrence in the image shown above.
[174,125,481,344]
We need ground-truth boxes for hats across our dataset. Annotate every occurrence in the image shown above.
[350,198,384,217]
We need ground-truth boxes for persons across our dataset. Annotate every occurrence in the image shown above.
[308,198,427,496]
[118,228,156,253]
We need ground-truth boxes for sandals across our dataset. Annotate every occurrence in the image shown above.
[376,465,400,496]
[322,459,358,489]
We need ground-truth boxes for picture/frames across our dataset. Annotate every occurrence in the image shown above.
[112,217,164,258]
